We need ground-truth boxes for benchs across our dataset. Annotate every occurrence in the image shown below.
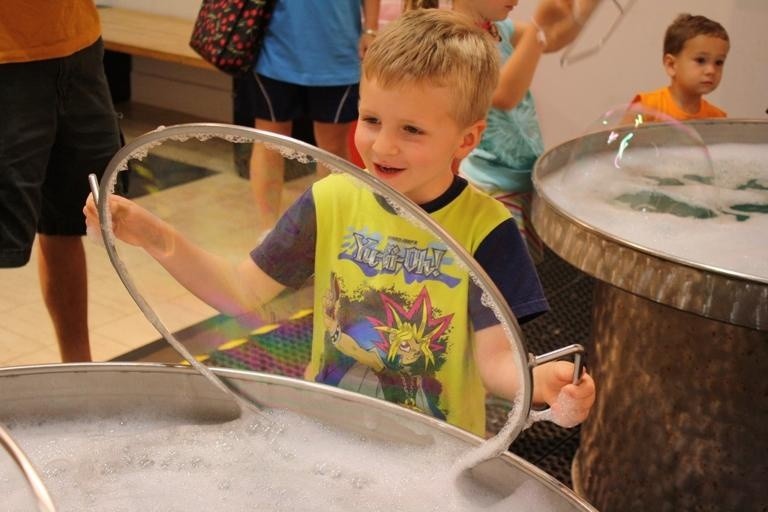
[92,4,319,182]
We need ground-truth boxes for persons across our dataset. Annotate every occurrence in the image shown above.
[396,1,605,266]
[616,12,732,130]
[83,7,597,460]
[234,1,382,244]
[0,0,129,366]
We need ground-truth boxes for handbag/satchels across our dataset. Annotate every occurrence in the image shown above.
[189,1,278,75]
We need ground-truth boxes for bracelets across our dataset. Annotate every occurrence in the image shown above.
[531,16,550,46]
[359,27,382,37]
